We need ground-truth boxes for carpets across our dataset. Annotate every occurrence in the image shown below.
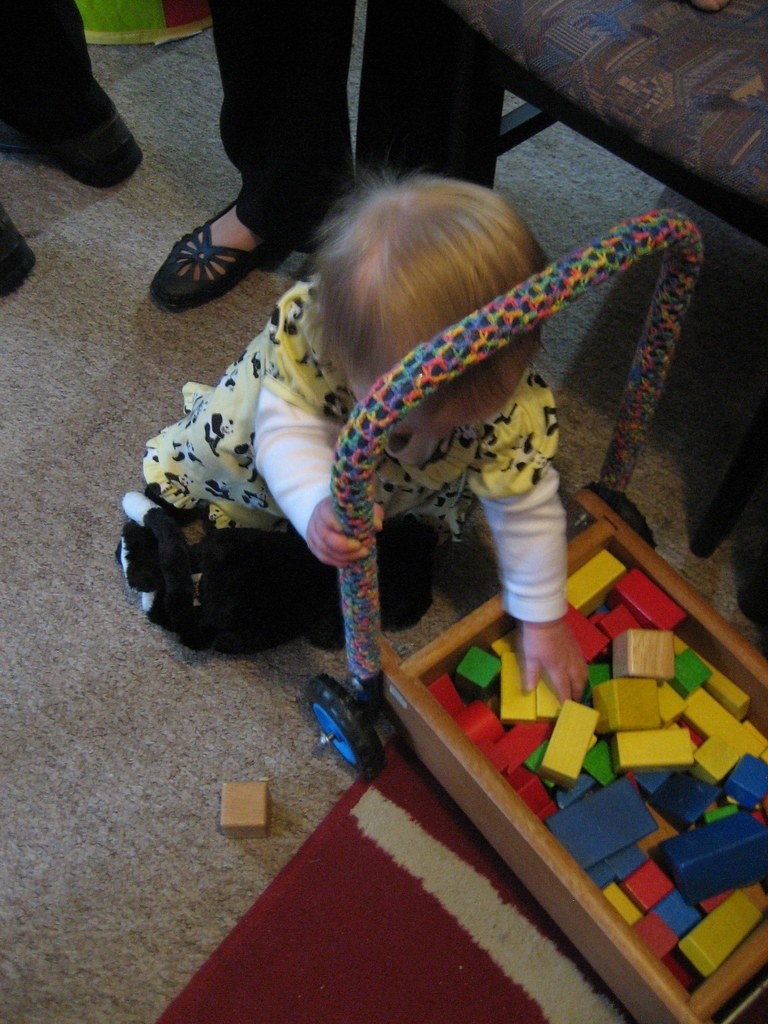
[155,734,636,1024]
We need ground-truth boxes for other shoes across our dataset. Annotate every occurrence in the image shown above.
[150,196,275,313]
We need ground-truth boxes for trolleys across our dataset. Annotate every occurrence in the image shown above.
[312,207,768,1024]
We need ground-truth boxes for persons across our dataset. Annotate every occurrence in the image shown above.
[0,0,142,302]
[117,166,588,706]
[151,0,508,316]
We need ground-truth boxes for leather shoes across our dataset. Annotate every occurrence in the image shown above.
[0,202,35,296]
[0,78,144,190]
